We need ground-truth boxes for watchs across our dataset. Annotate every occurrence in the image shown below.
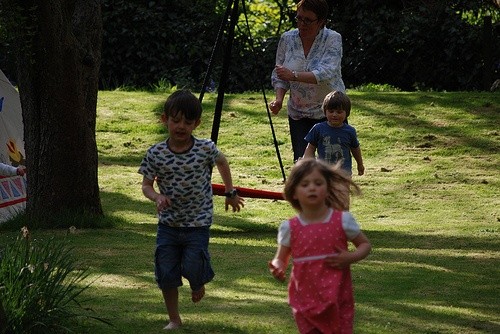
[224,189,237,197]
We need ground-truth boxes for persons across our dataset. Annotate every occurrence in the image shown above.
[268,0,347,165]
[137,89,245,330]
[302,91,365,179]
[268,157,371,334]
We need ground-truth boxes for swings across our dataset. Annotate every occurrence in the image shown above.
[211,0,286,201]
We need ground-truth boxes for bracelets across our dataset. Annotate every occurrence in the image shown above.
[292,70,298,81]
